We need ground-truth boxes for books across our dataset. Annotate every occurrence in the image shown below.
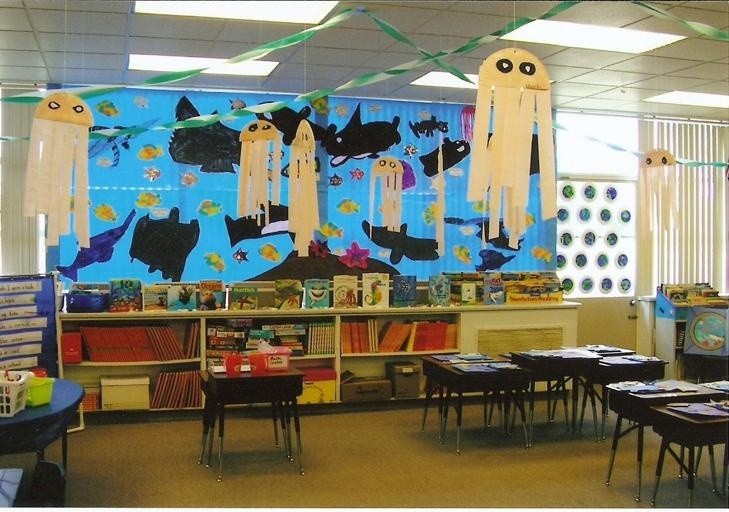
[107,278,329,312]
[363,273,389,309]
[76,381,101,410]
[150,368,202,408]
[432,345,729,417]
[206,319,335,358]
[462,282,475,305]
[394,275,417,307]
[479,272,504,305]
[428,275,452,307]
[333,275,358,309]
[341,320,457,353]
[660,282,728,356]
[80,320,200,362]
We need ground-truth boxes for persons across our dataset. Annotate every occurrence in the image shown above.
[465,289,473,299]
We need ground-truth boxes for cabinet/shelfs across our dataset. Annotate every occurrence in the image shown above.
[55,301,579,415]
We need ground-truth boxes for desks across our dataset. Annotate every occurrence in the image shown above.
[422,349,528,445]
[2,366,82,506]
[505,342,728,507]
[655,283,729,378]
[197,363,306,483]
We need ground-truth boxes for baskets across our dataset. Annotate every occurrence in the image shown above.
[0,369,35,419]
